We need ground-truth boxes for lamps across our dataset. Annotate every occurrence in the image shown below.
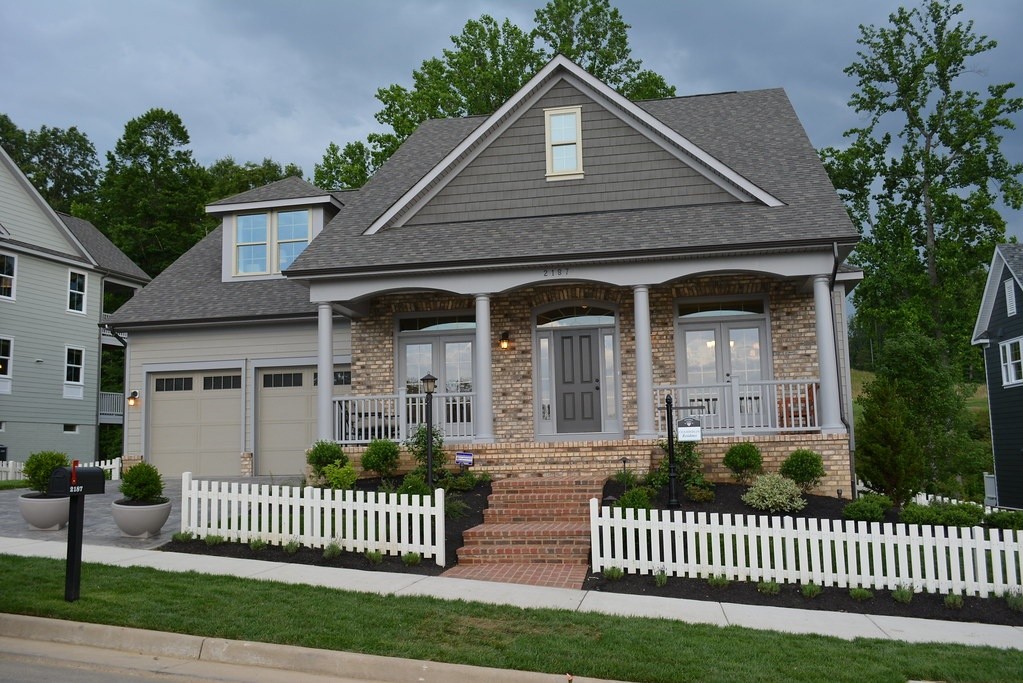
[127,390,139,405]
[499,333,509,350]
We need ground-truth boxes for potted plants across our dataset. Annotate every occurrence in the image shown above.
[16,450,70,533]
[110,462,173,538]
